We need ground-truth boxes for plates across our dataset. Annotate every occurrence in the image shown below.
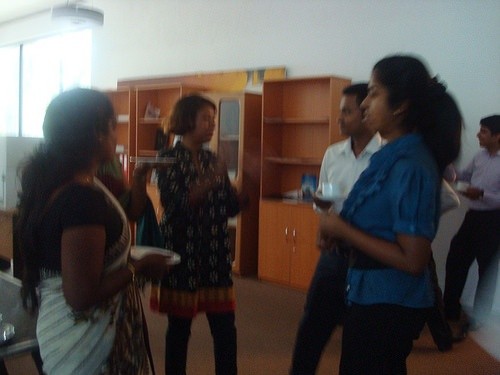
[130,245,181,267]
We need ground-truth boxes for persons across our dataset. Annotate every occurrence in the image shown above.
[417,177,466,351]
[316,56,461,375]
[288,84,391,375]
[152,95,256,375]
[440,115,500,327]
[19,89,173,375]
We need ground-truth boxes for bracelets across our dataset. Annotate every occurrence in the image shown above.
[479,191,484,200]
[128,262,137,281]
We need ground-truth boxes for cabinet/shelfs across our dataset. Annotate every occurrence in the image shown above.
[100,83,262,278]
[0,136,44,210]
[258,76,352,292]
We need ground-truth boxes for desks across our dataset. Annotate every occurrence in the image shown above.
[0,271,45,375]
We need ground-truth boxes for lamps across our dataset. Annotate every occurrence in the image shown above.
[0,8,104,48]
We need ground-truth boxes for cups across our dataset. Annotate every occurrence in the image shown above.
[456,181,470,193]
[313,182,335,215]
